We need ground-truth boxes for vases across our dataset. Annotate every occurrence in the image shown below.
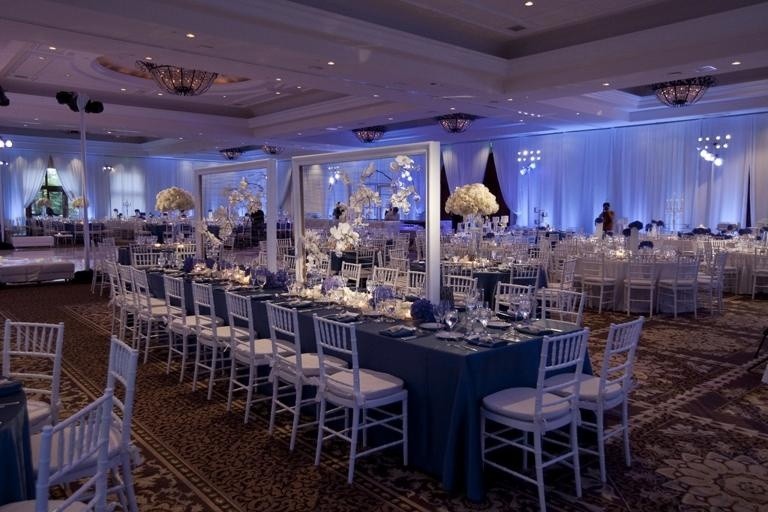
[78,209,84,221]
[41,205,46,215]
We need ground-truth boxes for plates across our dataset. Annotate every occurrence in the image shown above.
[419,322,445,329]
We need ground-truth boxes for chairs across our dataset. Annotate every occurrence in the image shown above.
[581,251,618,313]
[33,335,136,507]
[697,250,729,312]
[659,253,698,316]
[105,262,184,363]
[3,213,765,296]
[191,279,258,399]
[265,302,349,452]
[163,274,224,383]
[4,319,63,429]
[622,253,655,318]
[5,388,115,512]
[224,290,296,423]
[524,317,643,483]
[481,326,588,511]
[312,311,412,480]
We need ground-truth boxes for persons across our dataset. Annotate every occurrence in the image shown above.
[332,202,342,220]
[384,207,401,220]
[135,209,146,221]
[722,225,735,238]
[599,203,615,232]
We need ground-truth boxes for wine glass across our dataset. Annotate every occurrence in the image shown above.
[193,263,267,293]
[287,279,412,324]
[450,252,538,271]
[430,290,539,343]
[157,256,183,272]
[105,212,190,247]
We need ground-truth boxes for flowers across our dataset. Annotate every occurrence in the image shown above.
[71,196,89,209]
[37,198,52,208]
[156,187,195,213]
[444,183,499,218]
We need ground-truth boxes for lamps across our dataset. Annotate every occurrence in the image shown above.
[262,144,286,154]
[219,148,243,160]
[352,125,384,143]
[134,58,217,96]
[434,113,473,133]
[653,76,718,108]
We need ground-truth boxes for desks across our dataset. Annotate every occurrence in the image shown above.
[4,383,34,503]
